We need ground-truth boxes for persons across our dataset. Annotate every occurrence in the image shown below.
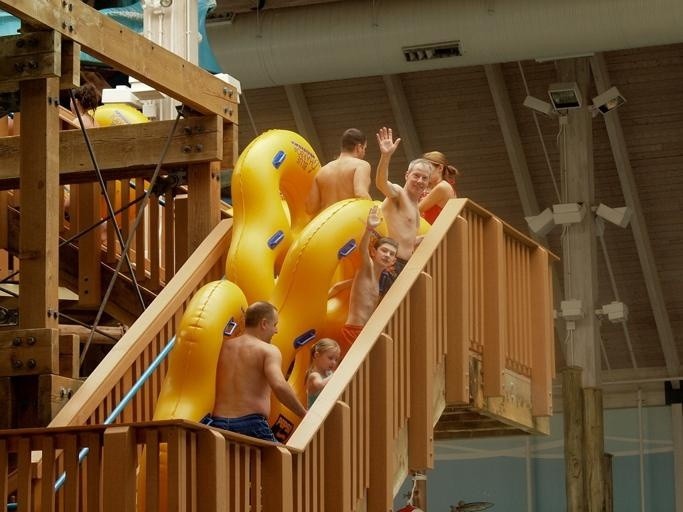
[371,125,432,289]
[298,128,375,216]
[415,150,459,227]
[66,82,102,130]
[202,300,307,442]
[340,204,399,360]
[301,336,340,409]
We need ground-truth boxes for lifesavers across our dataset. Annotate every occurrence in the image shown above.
[135,280,248,512]
[85,104,152,224]
[225,129,320,307]
[268,198,389,446]
